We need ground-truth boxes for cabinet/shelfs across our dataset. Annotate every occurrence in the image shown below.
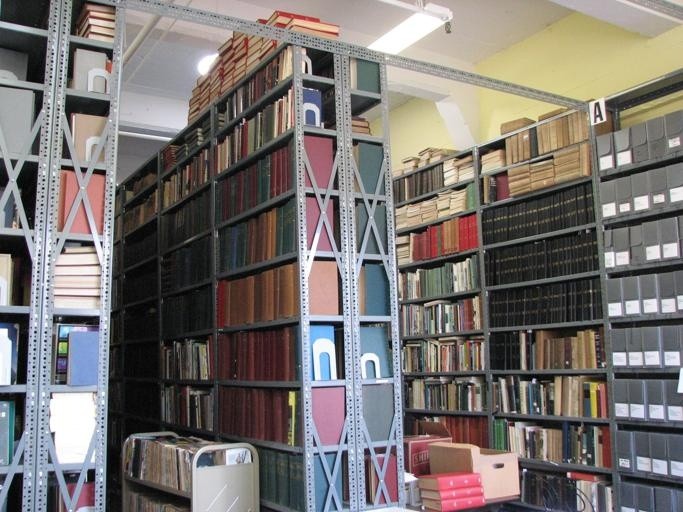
[0,0,683,512]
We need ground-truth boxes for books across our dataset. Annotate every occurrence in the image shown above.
[116,103,391,511]
[390,108,611,512]
[0,1,109,511]
[188,14,380,129]
